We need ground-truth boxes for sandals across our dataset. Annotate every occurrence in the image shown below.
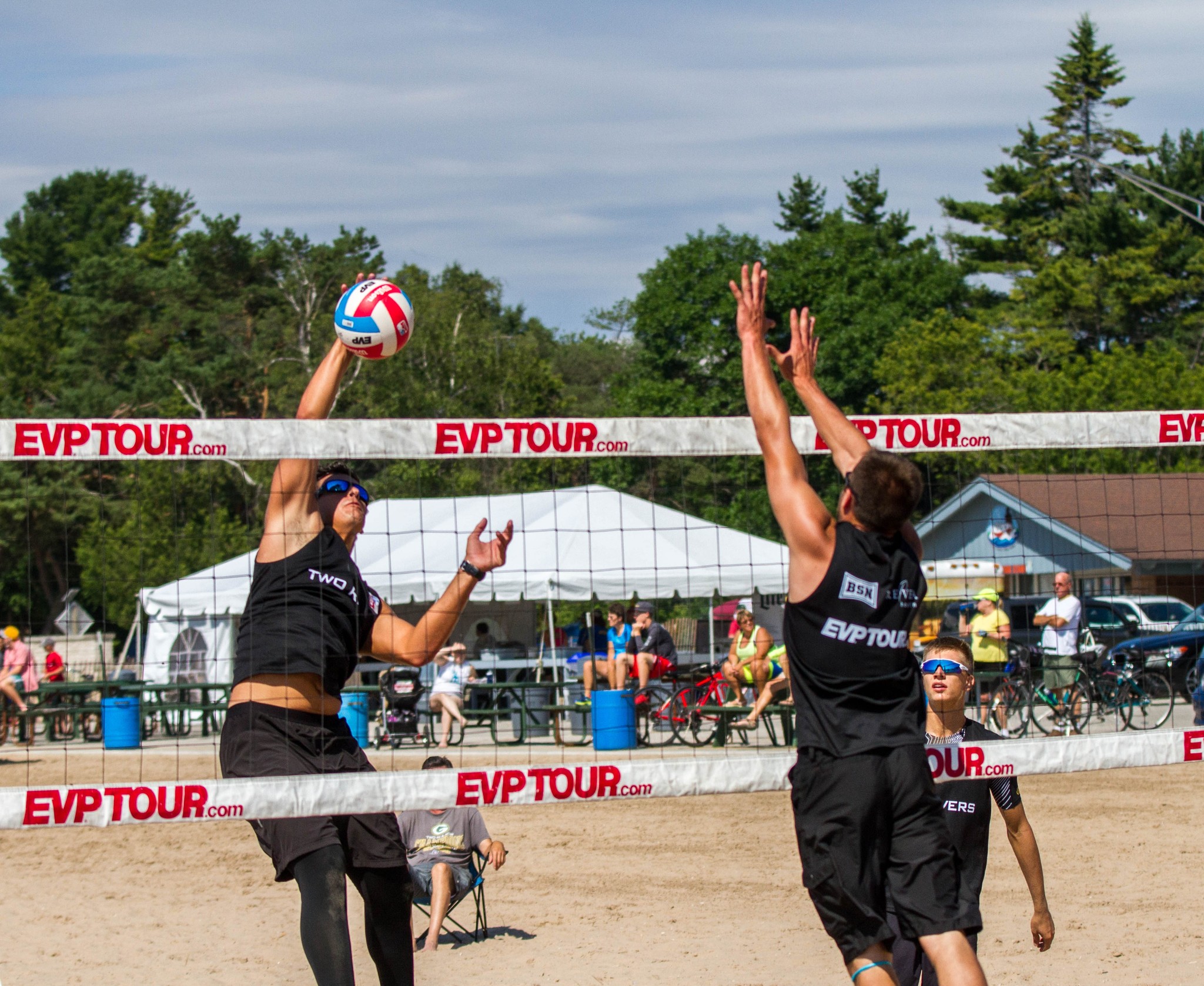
[723,699,747,707]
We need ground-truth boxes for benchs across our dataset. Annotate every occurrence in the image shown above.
[15,701,591,746]
[686,680,799,746]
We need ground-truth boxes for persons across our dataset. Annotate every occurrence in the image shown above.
[218,272,513,986]
[886,636,1054,986]
[0,572,1081,951]
[730,260,988,986]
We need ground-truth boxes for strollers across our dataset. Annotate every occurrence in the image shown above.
[374,663,430,750]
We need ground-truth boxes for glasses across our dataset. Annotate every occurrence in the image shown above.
[740,618,753,625]
[920,659,973,677]
[1052,582,1063,587]
[315,478,371,507]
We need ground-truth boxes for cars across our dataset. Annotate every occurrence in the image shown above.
[937,593,1204,705]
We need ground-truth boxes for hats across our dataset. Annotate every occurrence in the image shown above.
[39,638,55,648]
[632,602,655,615]
[973,587,1000,603]
[0,625,20,641]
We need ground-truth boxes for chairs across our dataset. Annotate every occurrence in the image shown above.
[412,848,509,944]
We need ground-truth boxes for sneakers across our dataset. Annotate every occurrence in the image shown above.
[575,693,592,705]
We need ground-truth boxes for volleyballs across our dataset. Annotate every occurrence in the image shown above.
[334,279,416,360]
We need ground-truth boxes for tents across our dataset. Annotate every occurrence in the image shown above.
[137,483,791,728]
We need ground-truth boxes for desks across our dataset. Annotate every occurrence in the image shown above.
[466,682,570,745]
[38,680,154,743]
[120,683,232,737]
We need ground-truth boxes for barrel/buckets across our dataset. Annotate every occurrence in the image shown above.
[337,693,370,749]
[592,689,639,750]
[508,687,550,737]
[567,683,609,734]
[650,682,691,732]
[101,697,141,749]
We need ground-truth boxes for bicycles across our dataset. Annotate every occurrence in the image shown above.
[634,657,779,748]
[1028,629,1176,737]
[0,671,179,748]
[990,636,1134,740]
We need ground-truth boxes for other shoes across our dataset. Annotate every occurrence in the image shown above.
[635,691,650,705]
[1041,729,1078,737]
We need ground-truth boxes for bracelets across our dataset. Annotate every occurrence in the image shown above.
[460,560,485,581]
